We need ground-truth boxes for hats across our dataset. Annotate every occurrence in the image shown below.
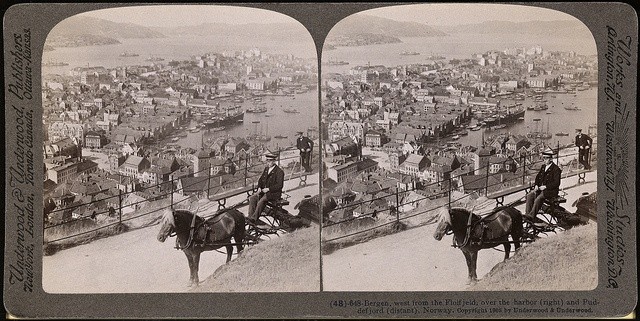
[575,128,582,132]
[295,132,304,136]
[541,152,556,158]
[266,155,276,160]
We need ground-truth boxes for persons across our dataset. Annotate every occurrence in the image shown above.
[524,151,561,219]
[246,153,284,221]
[574,128,593,169]
[295,130,314,172]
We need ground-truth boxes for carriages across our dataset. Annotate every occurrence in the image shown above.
[236,190,290,248]
[507,187,567,244]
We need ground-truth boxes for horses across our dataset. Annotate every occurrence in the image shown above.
[434,205,524,286]
[157,208,248,289]
